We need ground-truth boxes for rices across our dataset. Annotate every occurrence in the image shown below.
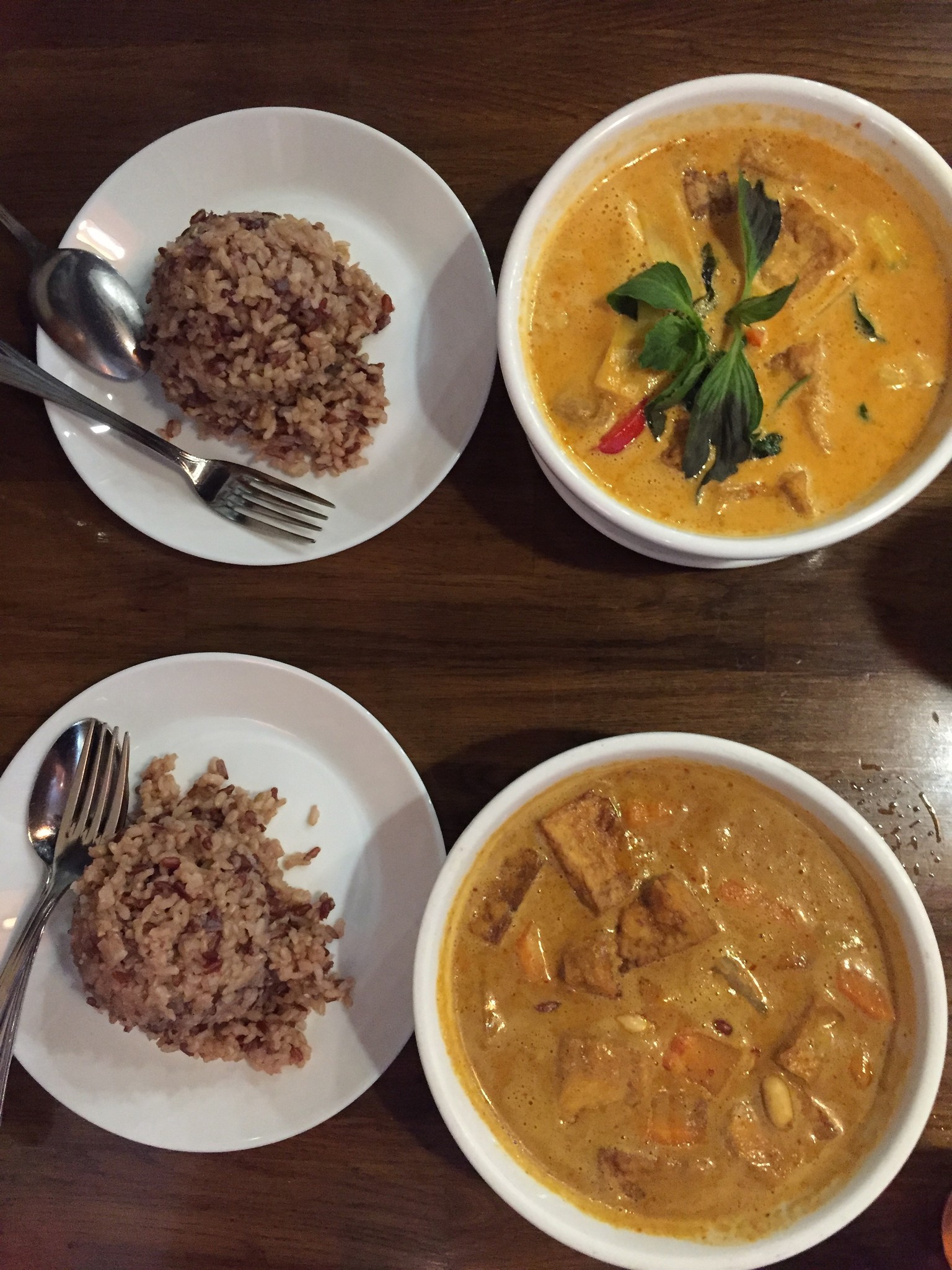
[63,750,366,1080]
[119,198,397,484]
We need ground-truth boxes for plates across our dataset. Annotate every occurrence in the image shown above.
[0,650,449,1152]
[37,107,500,569]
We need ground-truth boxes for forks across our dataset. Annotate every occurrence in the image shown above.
[0,722,132,1026]
[1,342,336,545]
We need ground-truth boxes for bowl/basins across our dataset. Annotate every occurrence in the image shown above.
[414,730,947,1270]
[495,69,951,569]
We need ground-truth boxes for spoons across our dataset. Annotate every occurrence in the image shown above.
[0,719,129,1139]
[1,209,163,380]
